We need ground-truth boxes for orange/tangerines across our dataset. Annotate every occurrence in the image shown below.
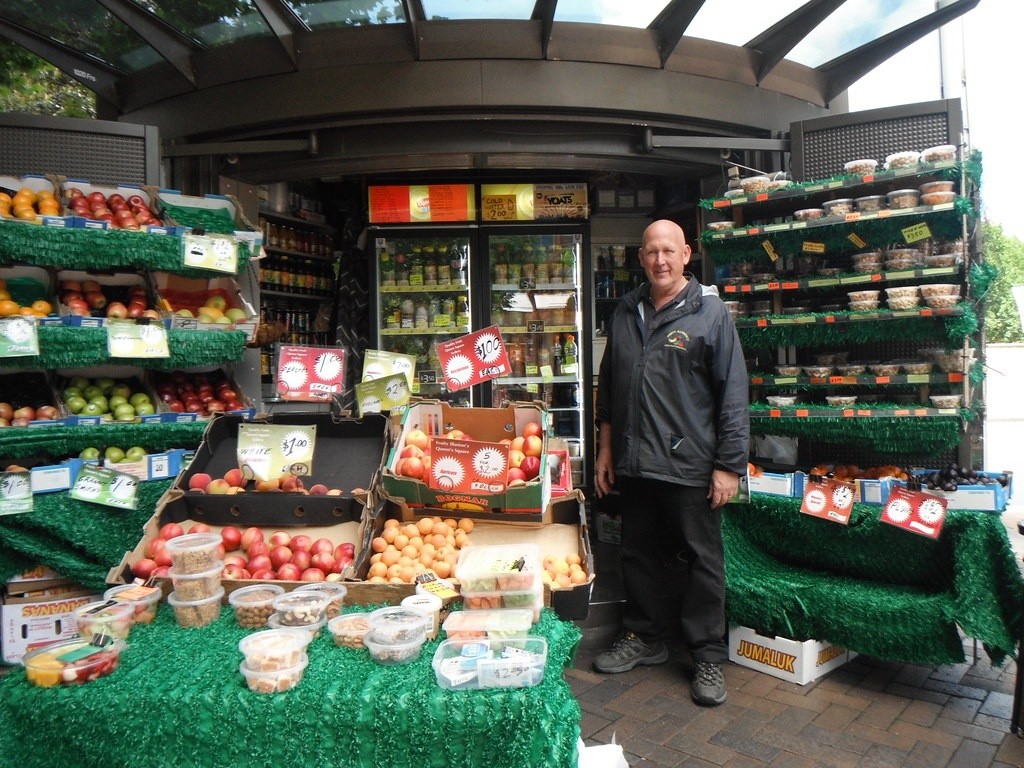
[0,187,60,221]
[0,277,53,318]
[810,462,908,482]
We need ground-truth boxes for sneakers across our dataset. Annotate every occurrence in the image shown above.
[690,661,726,705]
[592,632,669,673]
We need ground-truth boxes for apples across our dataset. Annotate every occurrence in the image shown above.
[0,372,242,475]
[60,279,160,319]
[63,187,162,230]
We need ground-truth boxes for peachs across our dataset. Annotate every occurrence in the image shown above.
[367,517,587,587]
[132,521,356,584]
[395,421,542,487]
[187,468,364,495]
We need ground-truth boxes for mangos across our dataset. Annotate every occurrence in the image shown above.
[174,294,246,324]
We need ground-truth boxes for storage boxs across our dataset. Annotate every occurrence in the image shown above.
[1,174,596,662]
[727,621,859,684]
[598,513,625,546]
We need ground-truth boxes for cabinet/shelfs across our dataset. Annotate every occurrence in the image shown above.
[700,146,973,432]
[253,209,336,376]
[591,267,703,378]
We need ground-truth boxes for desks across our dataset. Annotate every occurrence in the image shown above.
[714,491,1024,666]
[0,586,582,767]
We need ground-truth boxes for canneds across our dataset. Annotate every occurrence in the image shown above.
[260,306,312,332]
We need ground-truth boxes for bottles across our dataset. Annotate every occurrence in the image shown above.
[251,215,335,348]
[380,241,578,378]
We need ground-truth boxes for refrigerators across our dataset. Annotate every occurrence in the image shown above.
[367,176,598,498]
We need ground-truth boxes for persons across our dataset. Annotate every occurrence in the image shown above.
[591,220,750,704]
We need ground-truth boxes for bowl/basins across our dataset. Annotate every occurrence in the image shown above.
[706,144,964,409]
[20,531,548,695]
